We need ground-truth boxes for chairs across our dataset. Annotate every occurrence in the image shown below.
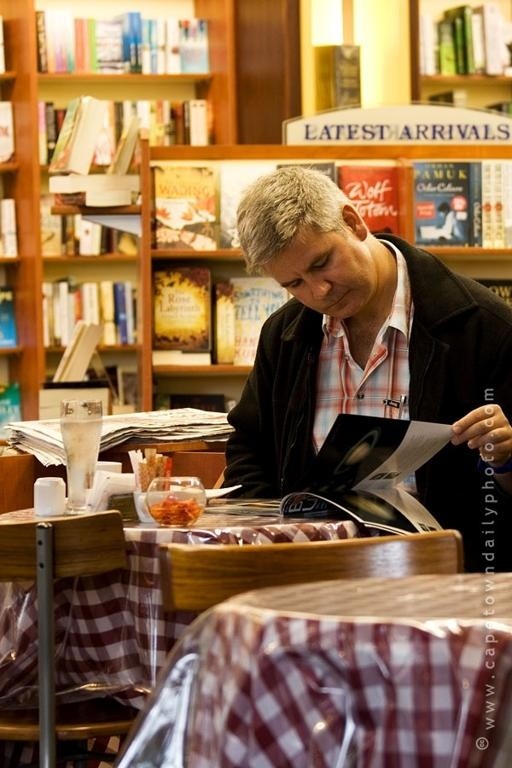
[0,427,464,768]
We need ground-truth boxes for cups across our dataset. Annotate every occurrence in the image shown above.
[134,492,157,524]
[33,477,66,517]
[58,399,102,517]
[95,461,123,474]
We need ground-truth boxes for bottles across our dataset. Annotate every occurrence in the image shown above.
[145,476,205,527]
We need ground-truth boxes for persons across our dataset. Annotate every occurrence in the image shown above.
[221,165,510,577]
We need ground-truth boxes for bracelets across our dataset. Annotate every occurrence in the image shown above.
[479,453,511,473]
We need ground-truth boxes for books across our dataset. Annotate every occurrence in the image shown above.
[203,411,460,536]
[416,6,512,115]
[1,10,297,429]
[281,163,510,308]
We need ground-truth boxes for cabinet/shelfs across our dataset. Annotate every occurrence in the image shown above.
[0,0,511,412]
